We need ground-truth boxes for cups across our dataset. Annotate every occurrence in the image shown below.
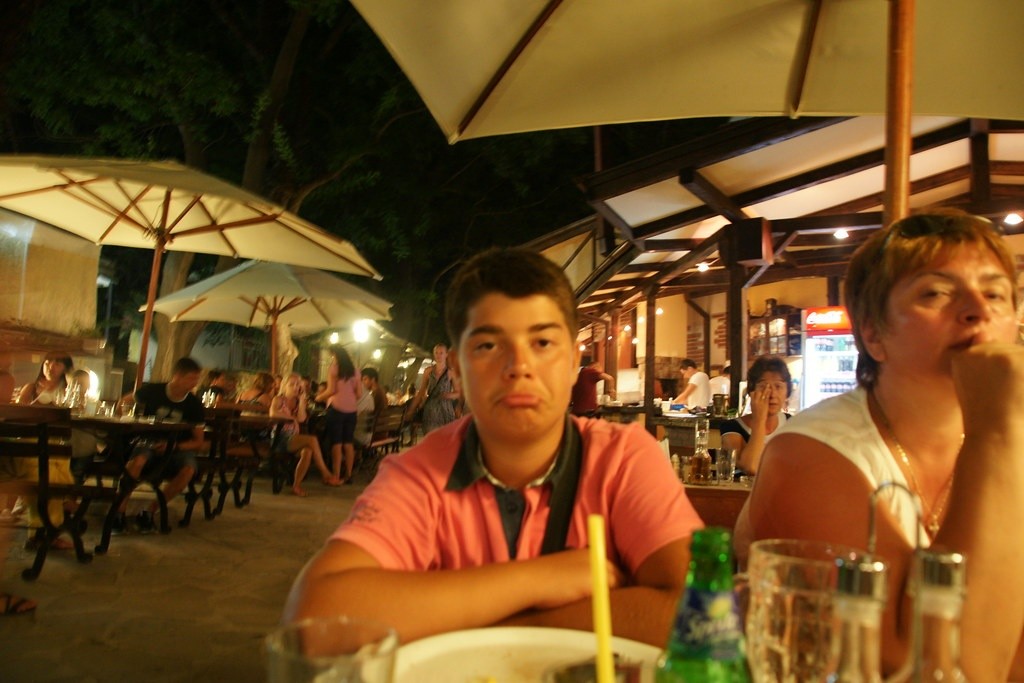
[101,401,116,418]
[712,393,729,418]
[715,447,737,487]
[660,400,670,412]
[602,395,610,405]
[260,616,398,683]
[121,399,136,423]
[743,536,871,683]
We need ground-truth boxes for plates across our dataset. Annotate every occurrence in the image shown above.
[314,623,663,683]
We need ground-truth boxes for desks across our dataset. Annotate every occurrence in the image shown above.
[73,405,187,553]
[603,400,737,456]
[671,467,755,497]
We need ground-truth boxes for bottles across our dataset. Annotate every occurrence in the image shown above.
[649,526,752,683]
[886,549,969,683]
[823,552,891,682]
[689,429,712,485]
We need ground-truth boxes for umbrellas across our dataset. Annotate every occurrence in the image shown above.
[138,260,394,376]
[350,0,1024,227]
[1,156,382,390]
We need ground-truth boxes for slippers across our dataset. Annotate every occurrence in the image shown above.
[0,590,37,615]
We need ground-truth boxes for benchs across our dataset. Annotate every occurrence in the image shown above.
[235,415,293,500]
[367,405,405,457]
[0,402,116,580]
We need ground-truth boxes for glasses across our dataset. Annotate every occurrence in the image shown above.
[756,380,786,389]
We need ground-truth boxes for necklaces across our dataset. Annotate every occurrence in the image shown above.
[870,389,964,538]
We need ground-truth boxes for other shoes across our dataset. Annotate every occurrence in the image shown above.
[322,476,343,487]
[132,513,154,534]
[290,488,308,498]
[110,511,126,538]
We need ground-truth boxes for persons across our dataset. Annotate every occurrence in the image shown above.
[0,351,98,547]
[733,208,1024,683]
[720,357,793,478]
[204,345,420,497]
[280,249,702,661]
[710,366,731,405]
[404,346,470,435]
[673,359,713,410]
[572,362,616,416]
[112,356,204,535]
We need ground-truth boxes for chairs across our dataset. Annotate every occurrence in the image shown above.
[198,406,234,519]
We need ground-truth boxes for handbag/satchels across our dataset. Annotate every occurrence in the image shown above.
[414,407,425,424]
